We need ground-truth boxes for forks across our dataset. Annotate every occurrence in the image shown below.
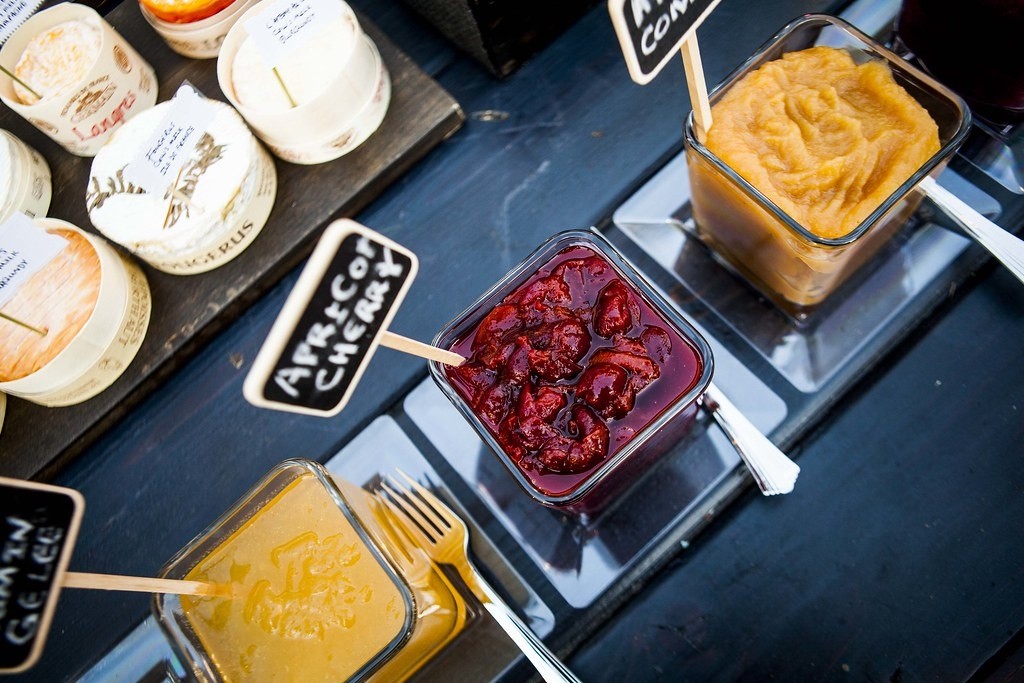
[374,468,584,683]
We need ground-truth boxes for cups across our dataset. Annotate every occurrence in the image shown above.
[427,228,714,518]
[152,458,477,683]
[684,13,974,327]
